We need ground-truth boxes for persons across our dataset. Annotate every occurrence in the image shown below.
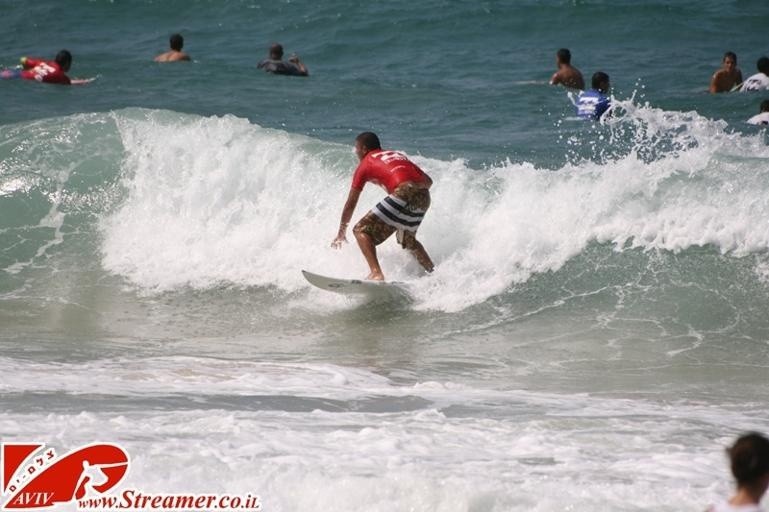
[2,48,97,88]
[327,131,435,283]
[258,43,308,78]
[701,430,769,512]
[153,32,190,64]
[710,50,742,95]
[547,47,583,92]
[575,71,614,121]
[748,101,769,126]
[735,56,769,94]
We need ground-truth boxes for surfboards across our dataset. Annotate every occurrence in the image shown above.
[302,268,413,296]
